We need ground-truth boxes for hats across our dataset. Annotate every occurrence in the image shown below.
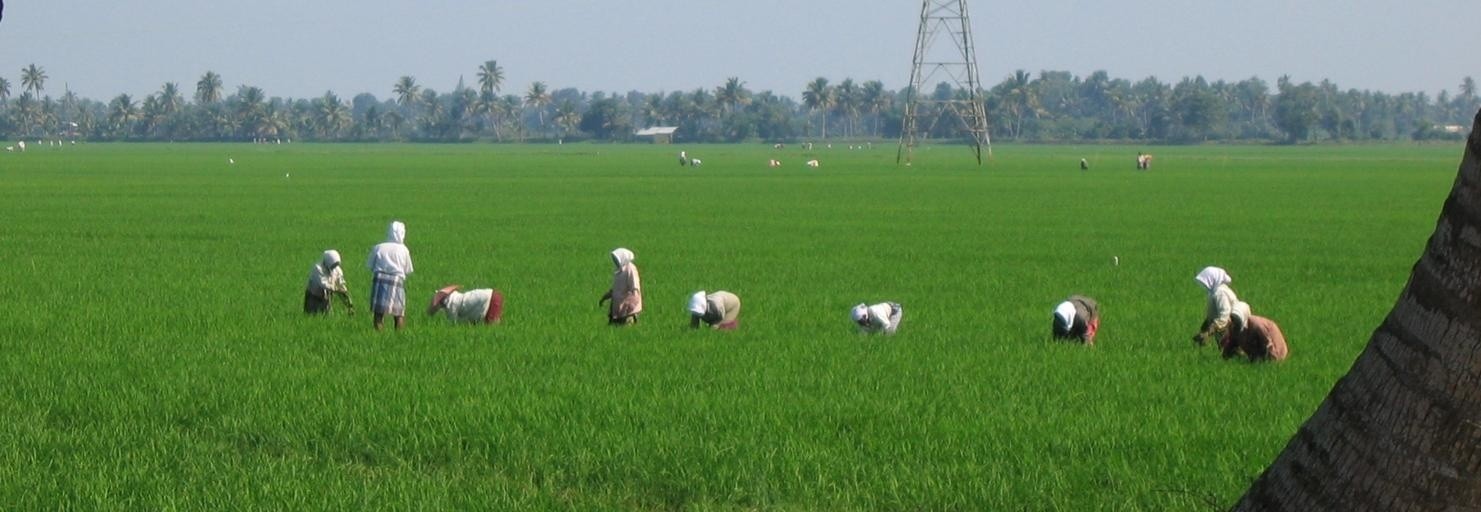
[428,286,462,315]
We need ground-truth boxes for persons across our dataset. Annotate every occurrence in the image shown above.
[368,221,414,331]
[1081,156,1090,171]
[7,139,76,151]
[769,159,780,166]
[1053,295,1100,347]
[1193,266,1239,353]
[1144,151,1152,167]
[807,160,819,167]
[775,142,870,151]
[1136,152,1145,169]
[851,302,902,334]
[681,151,701,165]
[687,290,741,329]
[1222,301,1288,362]
[254,138,291,143]
[304,249,355,317]
[441,287,503,325]
[599,246,643,326]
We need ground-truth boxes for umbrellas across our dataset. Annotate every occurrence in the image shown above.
[425,284,464,316]
